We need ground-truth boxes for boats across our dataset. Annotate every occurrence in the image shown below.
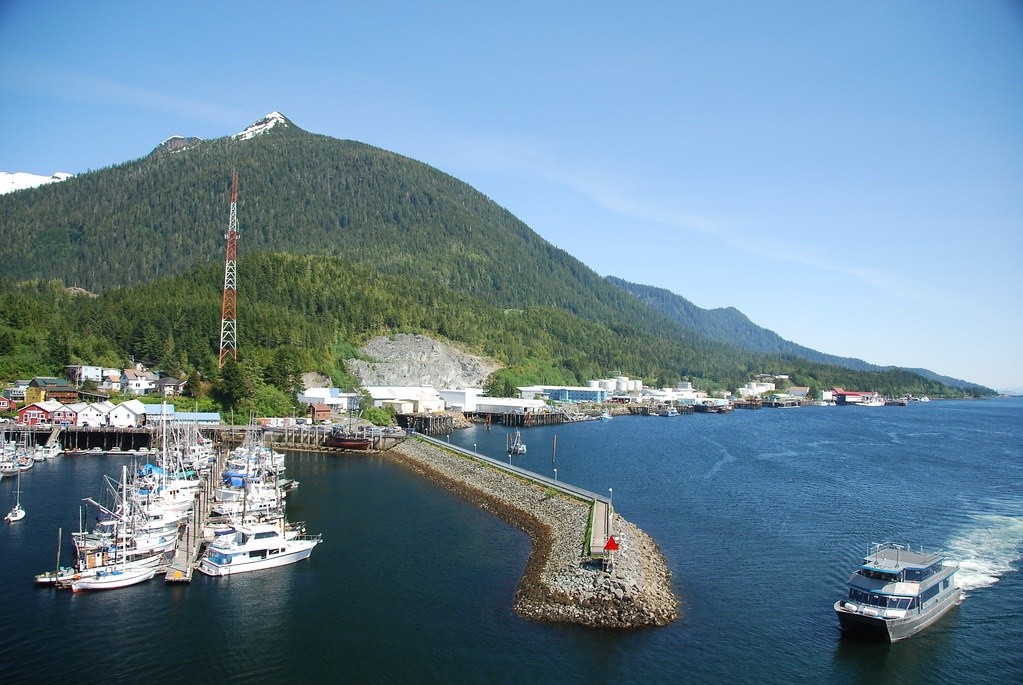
[855,394,930,407]
[834,539,961,644]
[326,433,371,450]
[509,442,527,455]
[777,397,802,409]
[659,400,680,417]
[600,410,614,421]
[814,400,837,406]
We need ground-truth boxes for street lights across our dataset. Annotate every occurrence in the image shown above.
[425,428,428,436]
[508,455,511,465]
[553,469,557,481]
[447,434,449,444]
[474,443,477,452]
[608,488,613,500]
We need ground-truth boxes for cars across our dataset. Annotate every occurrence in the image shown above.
[369,428,381,434]
[0,417,10,424]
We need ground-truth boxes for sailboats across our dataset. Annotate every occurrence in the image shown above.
[4,466,26,523]
[0,385,322,593]
